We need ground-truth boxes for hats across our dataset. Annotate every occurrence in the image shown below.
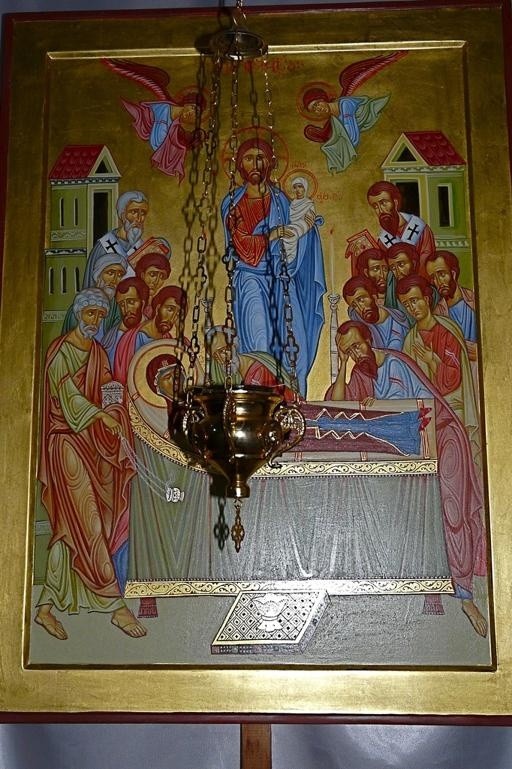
[295,81,339,123]
[220,124,291,189]
[281,168,319,203]
[172,84,217,127]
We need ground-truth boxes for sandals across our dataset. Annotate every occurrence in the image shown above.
[109,610,148,639]
[460,599,489,639]
[32,608,69,641]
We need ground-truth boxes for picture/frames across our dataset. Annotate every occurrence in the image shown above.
[0,0,510,725]
